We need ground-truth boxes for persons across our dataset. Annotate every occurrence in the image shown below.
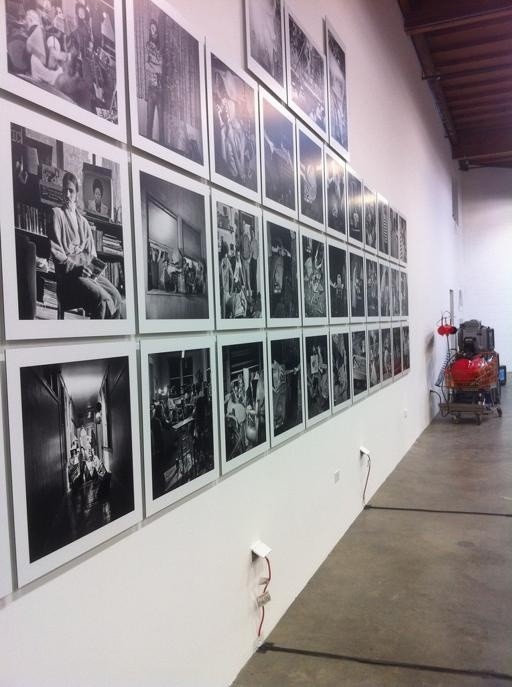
[215,68,352,451]
[49,172,126,319]
[88,179,109,218]
[143,19,165,146]
